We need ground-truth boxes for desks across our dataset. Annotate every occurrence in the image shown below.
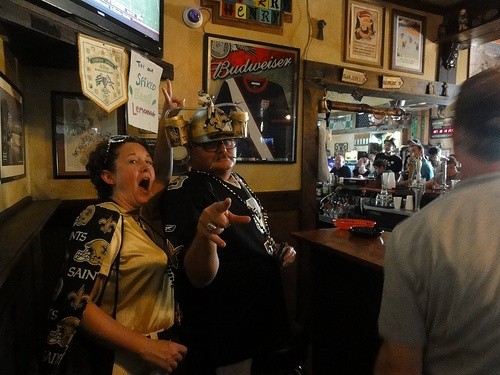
[290,227,392,323]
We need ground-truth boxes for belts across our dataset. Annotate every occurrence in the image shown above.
[147,325,174,341]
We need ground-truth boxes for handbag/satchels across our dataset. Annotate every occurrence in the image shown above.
[31,335,115,375]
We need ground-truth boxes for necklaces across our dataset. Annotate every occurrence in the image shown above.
[192,167,278,252]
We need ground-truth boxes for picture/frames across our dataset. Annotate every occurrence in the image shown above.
[202,32,301,164]
[389,8,426,76]
[50,90,128,179]
[0,71,27,184]
[343,0,387,68]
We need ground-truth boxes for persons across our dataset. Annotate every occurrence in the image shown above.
[351,135,463,197]
[50,79,188,375]
[328,154,352,179]
[371,62,500,375]
[154,90,331,375]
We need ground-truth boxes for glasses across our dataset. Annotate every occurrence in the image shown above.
[202,139,240,152]
[103,135,140,165]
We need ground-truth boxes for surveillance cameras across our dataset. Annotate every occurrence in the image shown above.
[183,8,203,28]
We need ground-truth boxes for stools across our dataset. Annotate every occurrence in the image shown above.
[250,322,310,375]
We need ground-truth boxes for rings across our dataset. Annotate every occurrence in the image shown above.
[205,222,216,233]
[289,261,293,264]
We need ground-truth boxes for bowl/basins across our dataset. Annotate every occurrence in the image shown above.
[348,226,384,239]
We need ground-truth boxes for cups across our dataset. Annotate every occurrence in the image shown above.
[415,178,425,211]
[375,184,393,207]
[452,180,460,190]
[434,161,446,192]
[408,156,422,189]
[405,195,413,210]
[316,172,375,220]
[393,197,402,210]
